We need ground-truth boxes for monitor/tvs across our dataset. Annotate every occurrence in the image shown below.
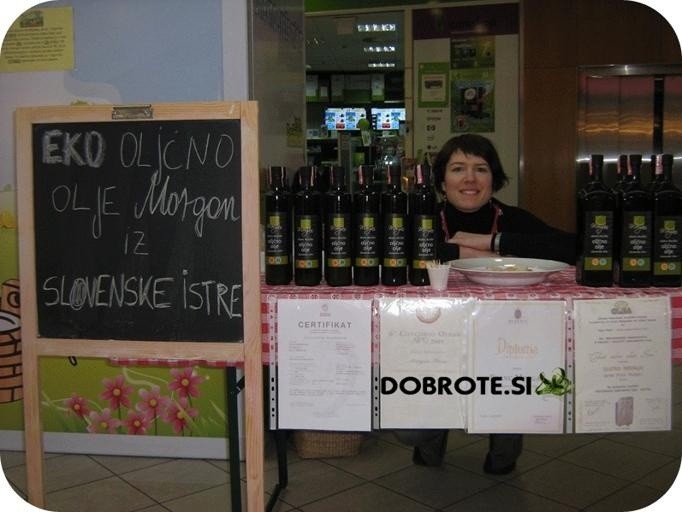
[323,105,369,132]
[369,104,406,130]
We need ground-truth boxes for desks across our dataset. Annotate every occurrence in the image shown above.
[224,262,681,512]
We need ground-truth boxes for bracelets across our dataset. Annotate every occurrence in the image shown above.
[493,229,502,255]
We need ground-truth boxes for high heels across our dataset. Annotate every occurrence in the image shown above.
[413,430,449,467]
[483,434,522,476]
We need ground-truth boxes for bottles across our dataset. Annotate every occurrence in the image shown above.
[651,155,682,287]
[294,166,324,284]
[351,164,382,288]
[320,166,352,287]
[577,154,611,290]
[648,156,659,184]
[614,155,651,288]
[263,166,289,287]
[379,164,408,287]
[410,162,441,284]
[577,160,591,189]
[615,156,633,183]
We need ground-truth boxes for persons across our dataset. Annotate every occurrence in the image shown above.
[412,133,579,475]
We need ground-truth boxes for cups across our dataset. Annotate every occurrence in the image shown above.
[426,264,451,292]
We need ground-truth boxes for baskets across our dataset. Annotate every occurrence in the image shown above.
[292,430,361,459]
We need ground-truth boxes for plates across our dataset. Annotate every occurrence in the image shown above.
[443,256,570,286]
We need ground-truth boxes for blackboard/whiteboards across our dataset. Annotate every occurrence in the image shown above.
[14,100,262,361]
